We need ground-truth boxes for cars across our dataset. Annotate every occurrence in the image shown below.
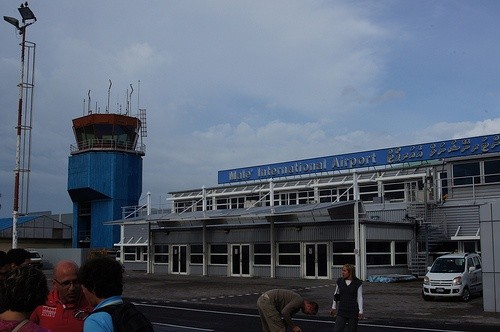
[28,250,43,267]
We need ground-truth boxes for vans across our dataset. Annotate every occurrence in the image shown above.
[422,252,482,302]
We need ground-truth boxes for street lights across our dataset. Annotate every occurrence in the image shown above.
[4,1,36,249]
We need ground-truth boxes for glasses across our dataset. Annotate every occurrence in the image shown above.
[54,279,81,287]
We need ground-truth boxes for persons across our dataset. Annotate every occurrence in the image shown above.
[31,260,95,332]
[77,256,153,332]
[0,265,50,332]
[257,289,319,332]
[0,248,32,280]
[331,264,364,332]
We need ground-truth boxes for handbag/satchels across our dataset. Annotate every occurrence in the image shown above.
[334,294,340,301]
